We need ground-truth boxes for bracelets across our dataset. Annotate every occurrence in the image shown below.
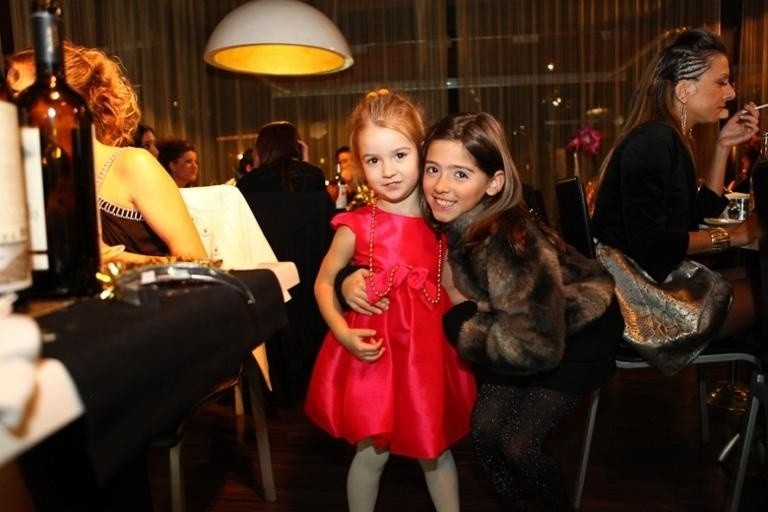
[707,224,731,255]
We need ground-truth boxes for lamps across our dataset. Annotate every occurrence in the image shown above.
[203,0,355,77]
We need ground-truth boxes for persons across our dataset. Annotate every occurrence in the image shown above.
[419,112,626,512]
[224,150,259,187]
[331,147,355,188]
[156,139,198,188]
[235,121,340,330]
[7,44,207,266]
[593,28,767,463]
[133,124,160,159]
[304,88,490,512]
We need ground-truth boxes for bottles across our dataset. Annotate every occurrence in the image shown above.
[18,1,99,297]
[332,161,348,214]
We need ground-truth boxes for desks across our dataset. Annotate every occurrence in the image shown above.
[0,252,283,512]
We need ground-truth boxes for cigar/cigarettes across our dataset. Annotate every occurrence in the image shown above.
[740,104,764,117]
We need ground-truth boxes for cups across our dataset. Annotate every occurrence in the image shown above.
[728,197,749,220]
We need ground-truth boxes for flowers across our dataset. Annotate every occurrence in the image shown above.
[565,128,601,154]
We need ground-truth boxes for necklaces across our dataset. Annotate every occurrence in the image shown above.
[369,196,443,303]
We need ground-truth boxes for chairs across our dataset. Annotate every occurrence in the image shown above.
[558,176,760,512]
[170,354,277,512]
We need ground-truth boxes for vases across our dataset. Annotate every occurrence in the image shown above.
[573,153,579,176]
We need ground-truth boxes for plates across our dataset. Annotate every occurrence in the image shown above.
[703,218,742,225]
